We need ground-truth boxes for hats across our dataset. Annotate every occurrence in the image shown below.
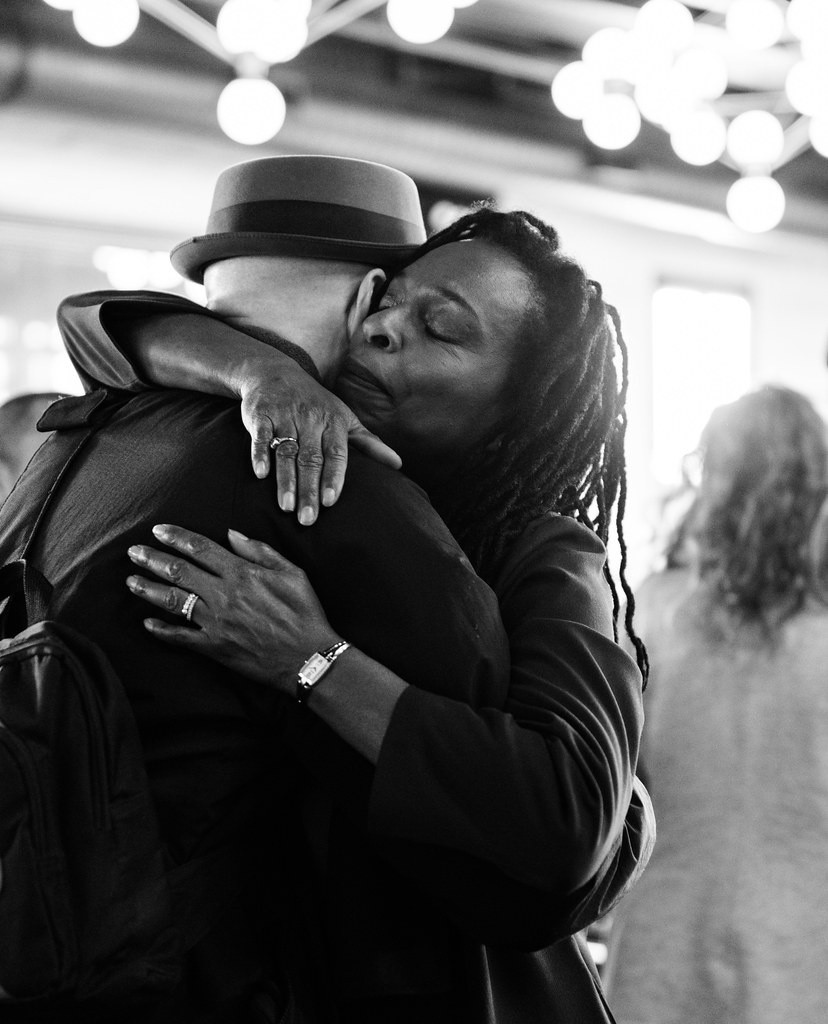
[170,157,430,284]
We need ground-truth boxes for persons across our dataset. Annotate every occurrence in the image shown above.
[58,206,659,1024]
[590,380,826,1024]
[0,157,657,1024]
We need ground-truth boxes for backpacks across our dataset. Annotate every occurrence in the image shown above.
[2,389,166,1005]
